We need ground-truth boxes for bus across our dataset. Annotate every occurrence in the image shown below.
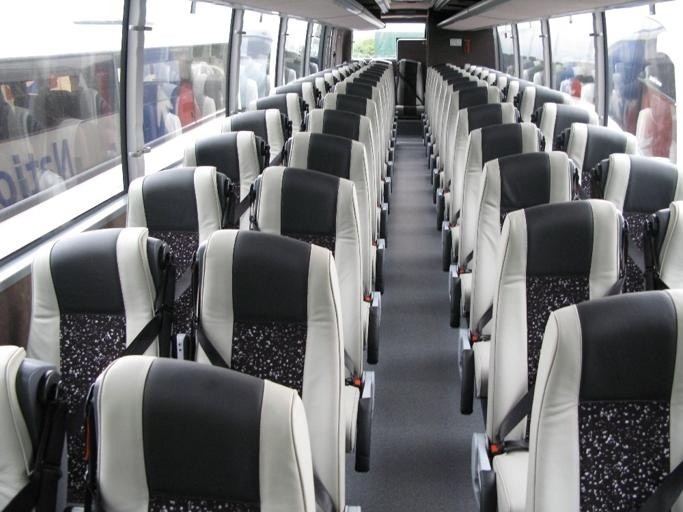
[0,0,320,221]
[498,1,676,170]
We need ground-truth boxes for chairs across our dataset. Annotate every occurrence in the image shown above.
[2,55,395,512]
[397,60,425,117]
[422,60,683,512]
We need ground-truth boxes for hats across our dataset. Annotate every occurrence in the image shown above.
[635,51,677,105]
